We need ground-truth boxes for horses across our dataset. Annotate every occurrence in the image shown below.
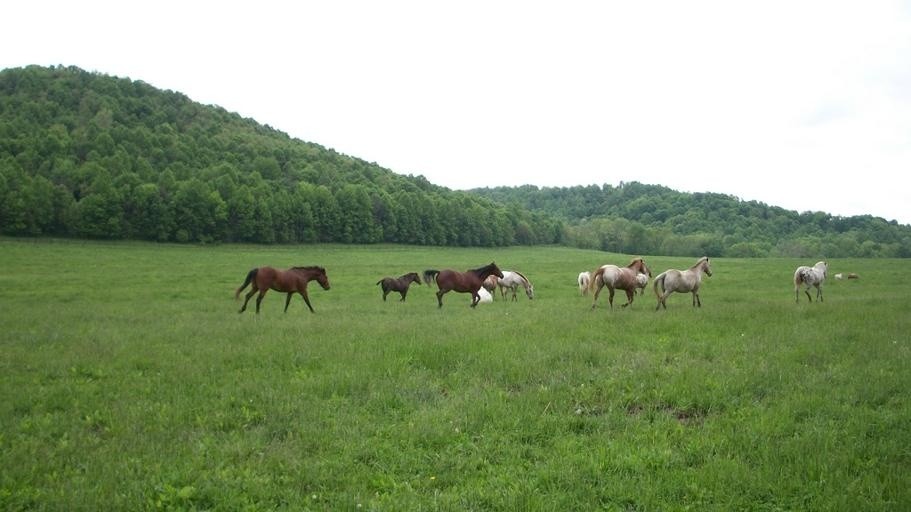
[376,272,421,302]
[653,256,713,311]
[235,264,331,313]
[794,261,828,304]
[577,257,652,313]
[423,260,534,309]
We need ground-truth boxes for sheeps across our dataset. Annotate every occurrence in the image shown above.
[848,273,858,279]
[834,273,843,281]
